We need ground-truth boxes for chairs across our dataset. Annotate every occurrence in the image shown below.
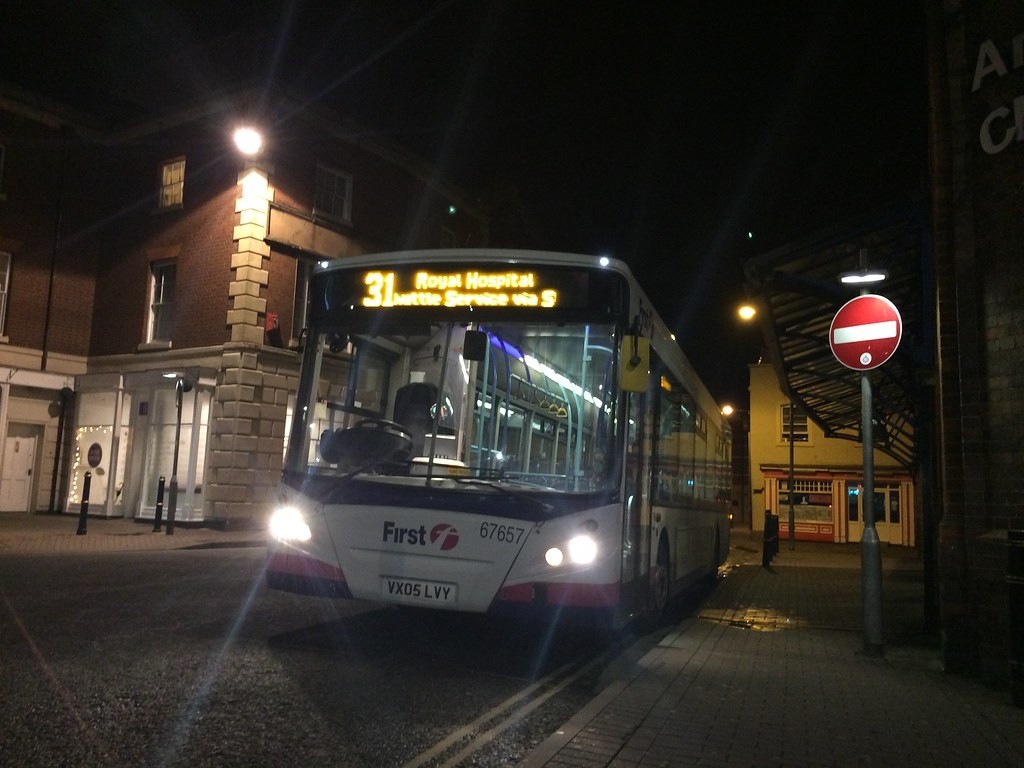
[394,383,453,457]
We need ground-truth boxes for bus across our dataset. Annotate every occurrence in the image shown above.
[266,248,734,635]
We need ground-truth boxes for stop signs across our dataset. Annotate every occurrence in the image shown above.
[829,295,903,371]
